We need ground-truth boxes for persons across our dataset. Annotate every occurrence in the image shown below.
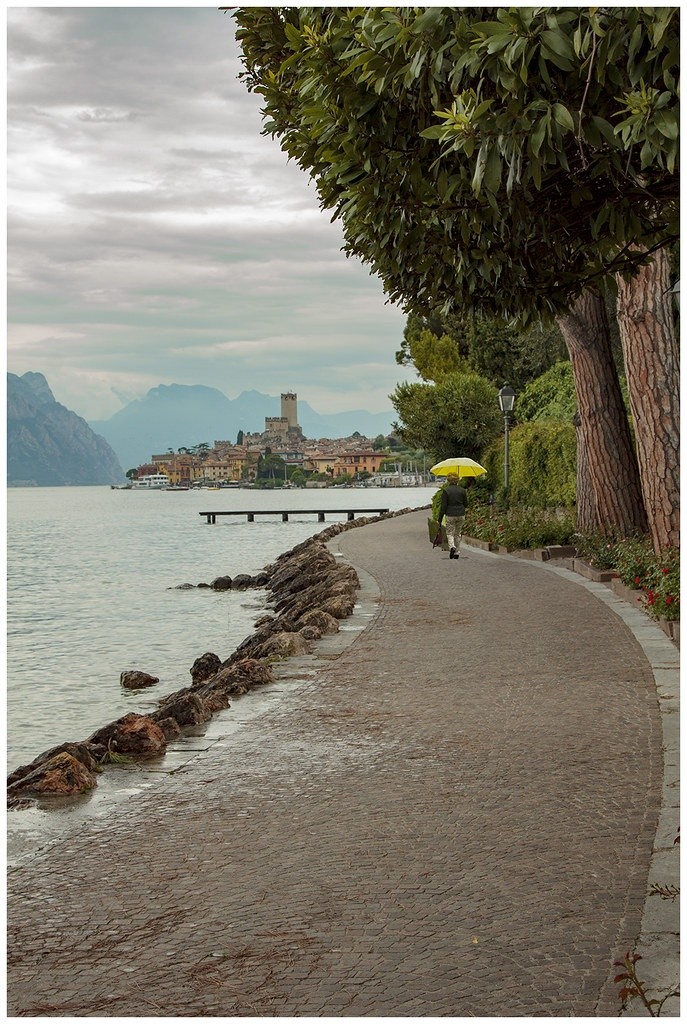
[437,471,467,560]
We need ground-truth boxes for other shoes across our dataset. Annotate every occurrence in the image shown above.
[450,547,456,559]
[453,555,459,559]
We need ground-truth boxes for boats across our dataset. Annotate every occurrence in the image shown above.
[192,485,220,490]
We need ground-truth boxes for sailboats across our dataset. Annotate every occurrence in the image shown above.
[165,459,190,491]
[273,465,294,489]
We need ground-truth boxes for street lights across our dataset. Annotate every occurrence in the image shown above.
[494,380,520,515]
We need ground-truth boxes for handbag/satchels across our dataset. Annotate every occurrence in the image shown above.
[433,526,442,549]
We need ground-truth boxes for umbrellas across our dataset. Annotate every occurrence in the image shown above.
[430,456,488,477]
